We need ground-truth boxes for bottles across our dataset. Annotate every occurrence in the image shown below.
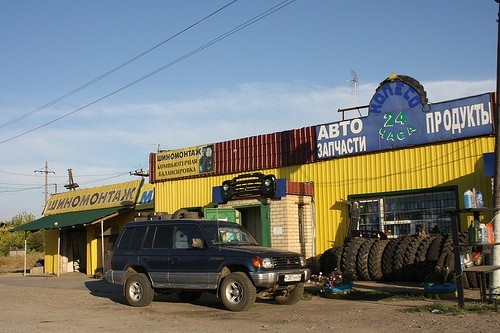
[485,223,495,243]
[477,192,483,208]
[478,224,487,243]
[464,189,474,208]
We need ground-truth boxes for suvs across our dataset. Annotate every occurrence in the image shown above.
[104,219,312,312]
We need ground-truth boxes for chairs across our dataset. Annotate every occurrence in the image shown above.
[175,230,193,249]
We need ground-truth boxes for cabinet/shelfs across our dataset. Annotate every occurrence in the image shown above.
[346,184,500,306]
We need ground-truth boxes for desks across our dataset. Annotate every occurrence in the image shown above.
[463,264,500,304]
